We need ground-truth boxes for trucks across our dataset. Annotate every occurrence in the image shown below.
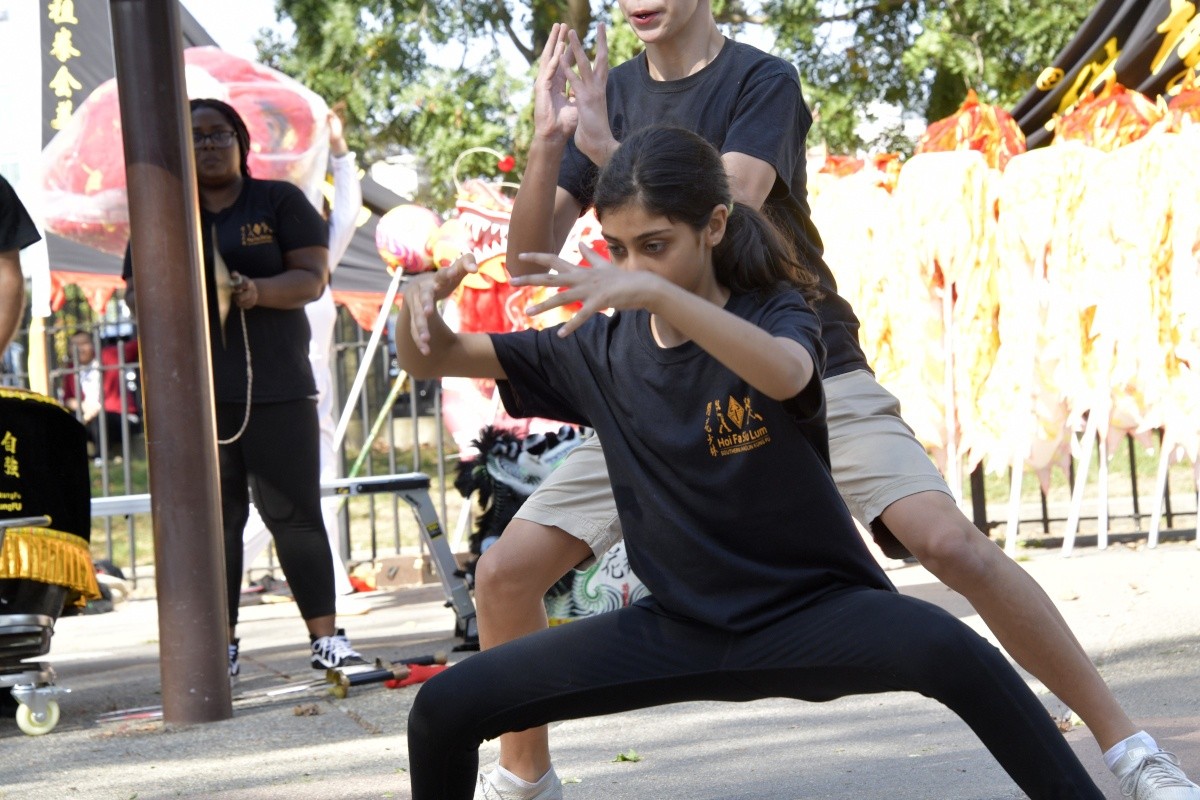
[333,303,443,419]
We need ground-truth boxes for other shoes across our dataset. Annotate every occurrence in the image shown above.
[93,450,123,468]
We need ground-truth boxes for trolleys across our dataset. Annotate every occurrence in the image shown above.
[0,515,72,736]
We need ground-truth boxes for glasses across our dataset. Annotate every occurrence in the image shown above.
[193,130,238,149]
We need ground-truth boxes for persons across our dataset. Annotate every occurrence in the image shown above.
[121,99,371,687]
[0,175,42,357]
[396,0,1200,800]
[63,330,135,467]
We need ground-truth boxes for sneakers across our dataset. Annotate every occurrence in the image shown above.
[310,629,370,669]
[228,639,240,677]
[1118,749,1200,800]
[470,763,563,800]
